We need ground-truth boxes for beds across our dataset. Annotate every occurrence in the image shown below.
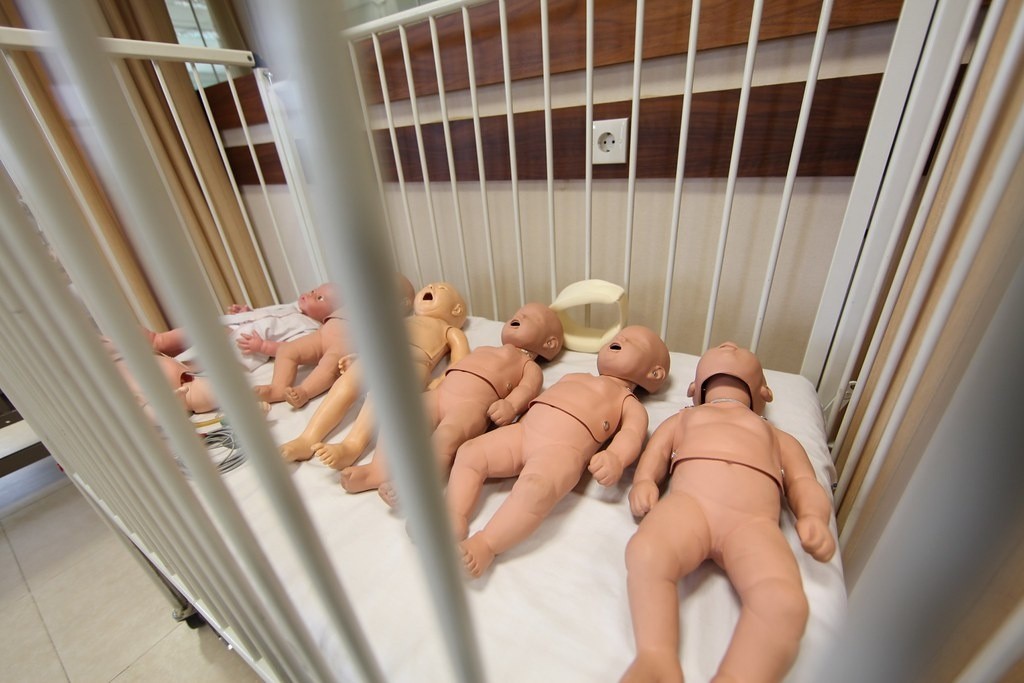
[1,0,1024,683]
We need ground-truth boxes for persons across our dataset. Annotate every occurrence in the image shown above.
[627,339,838,683]
[97,272,669,584]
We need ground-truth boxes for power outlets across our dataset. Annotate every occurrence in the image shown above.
[590,117,628,165]
